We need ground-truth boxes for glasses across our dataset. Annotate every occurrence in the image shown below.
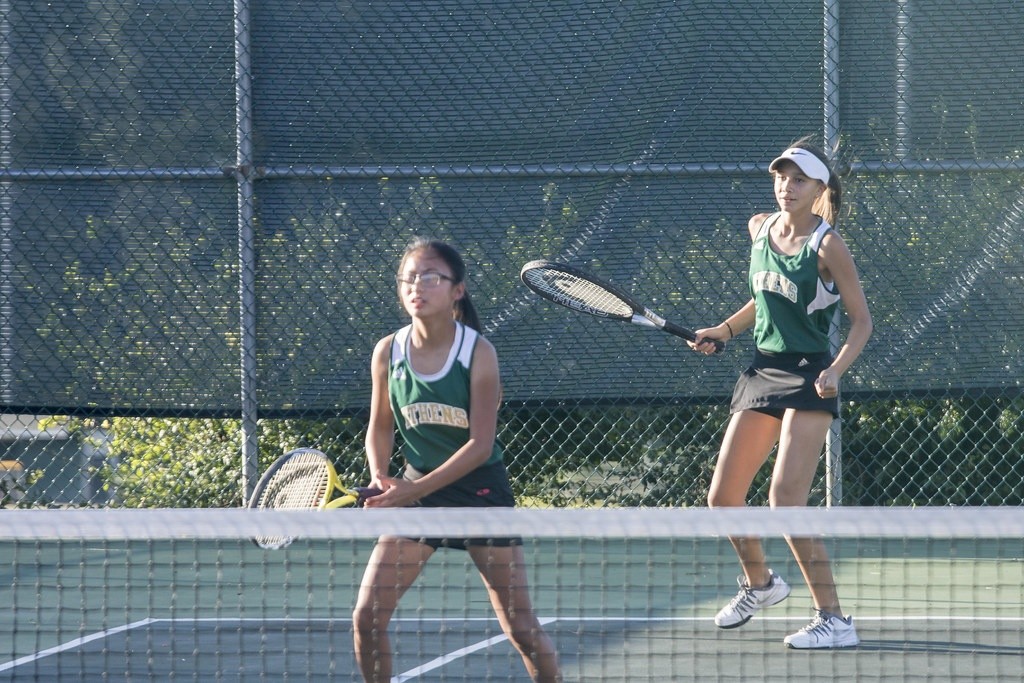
[394,271,458,288]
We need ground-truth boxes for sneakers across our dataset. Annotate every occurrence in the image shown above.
[714,568,791,630]
[784,607,860,650]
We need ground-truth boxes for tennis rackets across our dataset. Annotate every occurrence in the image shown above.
[244,447,383,553]
[520,257,729,357]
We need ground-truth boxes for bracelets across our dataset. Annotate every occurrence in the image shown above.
[723,321,733,338]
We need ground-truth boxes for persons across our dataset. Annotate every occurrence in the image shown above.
[686,141,873,649]
[353,240,564,683]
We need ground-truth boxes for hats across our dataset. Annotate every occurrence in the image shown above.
[769,147,830,186]
